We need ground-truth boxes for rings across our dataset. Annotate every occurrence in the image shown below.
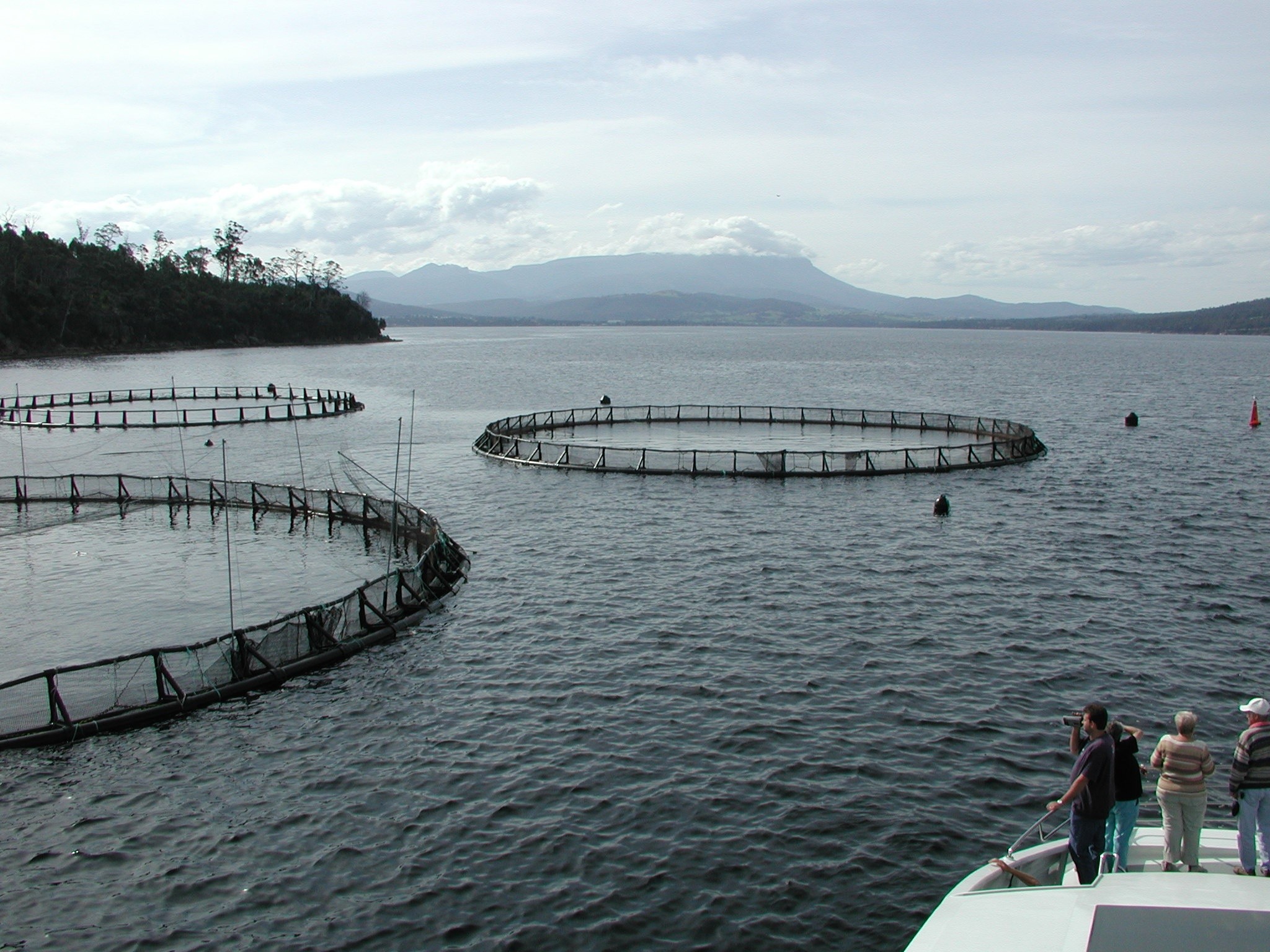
[1050,806,1051,808]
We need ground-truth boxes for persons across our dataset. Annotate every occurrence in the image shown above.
[1229,698,1270,877]
[1150,710,1215,873]
[1102,722,1145,872]
[1046,700,1115,885]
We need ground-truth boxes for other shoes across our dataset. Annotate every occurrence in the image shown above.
[1234,866,1256,876]
[1161,860,1171,871]
[1261,871,1270,876]
[1189,866,1207,872]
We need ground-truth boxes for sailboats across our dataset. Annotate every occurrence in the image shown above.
[1248,395,1261,428]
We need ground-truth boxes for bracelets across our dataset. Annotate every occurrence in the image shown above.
[1057,799,1065,806]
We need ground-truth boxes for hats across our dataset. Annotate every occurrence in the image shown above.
[1239,697,1270,716]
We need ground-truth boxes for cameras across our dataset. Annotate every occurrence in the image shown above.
[1063,715,1083,728]
